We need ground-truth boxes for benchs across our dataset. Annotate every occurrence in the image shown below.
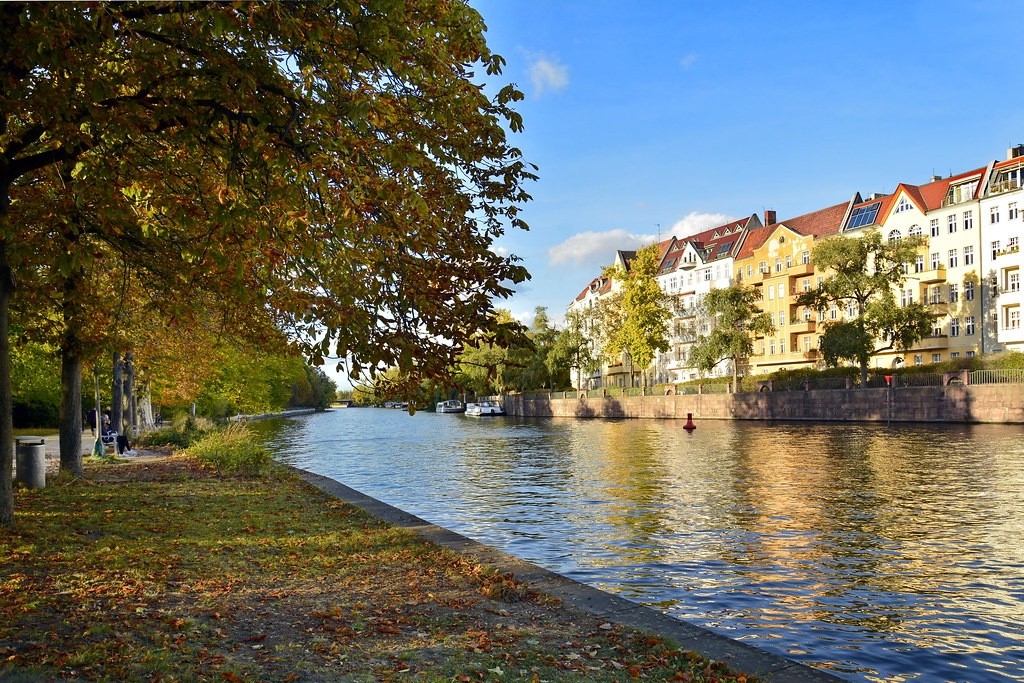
[101,435,120,454]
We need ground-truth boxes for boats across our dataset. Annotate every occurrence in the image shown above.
[436,399,465,413]
[464,401,505,417]
[385,401,409,410]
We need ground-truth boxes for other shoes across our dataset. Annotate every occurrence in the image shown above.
[119,452,129,457]
[127,449,138,455]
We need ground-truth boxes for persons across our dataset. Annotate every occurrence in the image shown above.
[82,405,137,457]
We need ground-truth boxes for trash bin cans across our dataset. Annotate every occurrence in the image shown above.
[14,436,46,490]
